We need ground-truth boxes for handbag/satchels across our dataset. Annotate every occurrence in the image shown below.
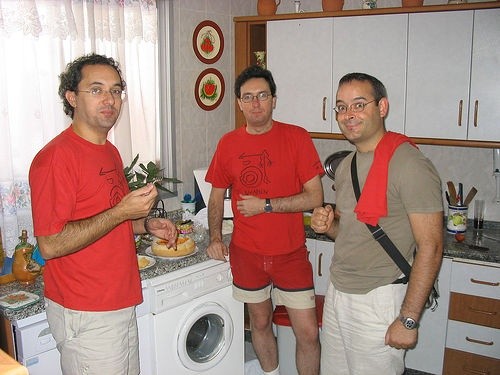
[424,278,441,309]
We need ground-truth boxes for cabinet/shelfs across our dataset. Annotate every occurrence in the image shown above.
[406,256,499,375]
[266,13,408,135]
[405,8,500,144]
[251,238,336,375]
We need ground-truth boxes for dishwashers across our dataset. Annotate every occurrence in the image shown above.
[11,287,156,374]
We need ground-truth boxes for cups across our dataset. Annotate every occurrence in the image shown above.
[473,200,486,229]
[180,200,196,221]
[445,204,468,234]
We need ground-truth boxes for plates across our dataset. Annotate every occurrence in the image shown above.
[136,255,156,271]
[194,68,225,110]
[193,21,224,64]
[145,246,199,260]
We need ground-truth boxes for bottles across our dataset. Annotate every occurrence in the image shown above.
[361,0,378,9]
[322,0,345,12]
[253,50,268,68]
[256,0,281,16]
[401,0,425,7]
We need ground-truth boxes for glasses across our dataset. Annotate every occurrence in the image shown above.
[240,92,271,102]
[78,86,126,97]
[333,100,376,113]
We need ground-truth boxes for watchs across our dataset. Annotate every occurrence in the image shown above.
[398,316,419,329]
[265,199,272,213]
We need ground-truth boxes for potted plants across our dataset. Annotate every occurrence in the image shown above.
[123,154,183,248]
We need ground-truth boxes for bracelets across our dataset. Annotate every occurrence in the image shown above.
[145,217,151,233]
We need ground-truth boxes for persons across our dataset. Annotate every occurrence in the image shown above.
[29,55,178,375]
[207,67,324,375]
[311,72,444,375]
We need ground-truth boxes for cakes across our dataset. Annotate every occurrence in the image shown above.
[151,236,195,257]
[139,257,149,267]
